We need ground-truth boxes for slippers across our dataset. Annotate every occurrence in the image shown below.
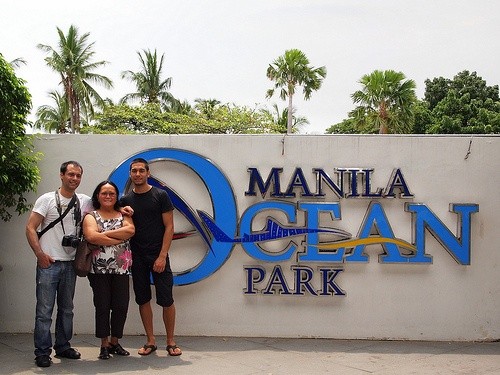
[166,345,182,356]
[138,344,157,355]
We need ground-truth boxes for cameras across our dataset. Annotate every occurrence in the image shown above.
[62,235,81,248]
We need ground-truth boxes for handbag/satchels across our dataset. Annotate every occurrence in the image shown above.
[73,240,92,277]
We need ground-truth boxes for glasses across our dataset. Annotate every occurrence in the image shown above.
[99,192,117,197]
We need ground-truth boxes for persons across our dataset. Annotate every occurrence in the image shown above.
[26,161,134,367]
[116,158,182,356]
[82,181,135,360]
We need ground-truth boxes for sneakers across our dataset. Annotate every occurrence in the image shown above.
[57,348,81,359]
[34,355,51,367]
[99,345,110,360]
[109,343,130,356]
